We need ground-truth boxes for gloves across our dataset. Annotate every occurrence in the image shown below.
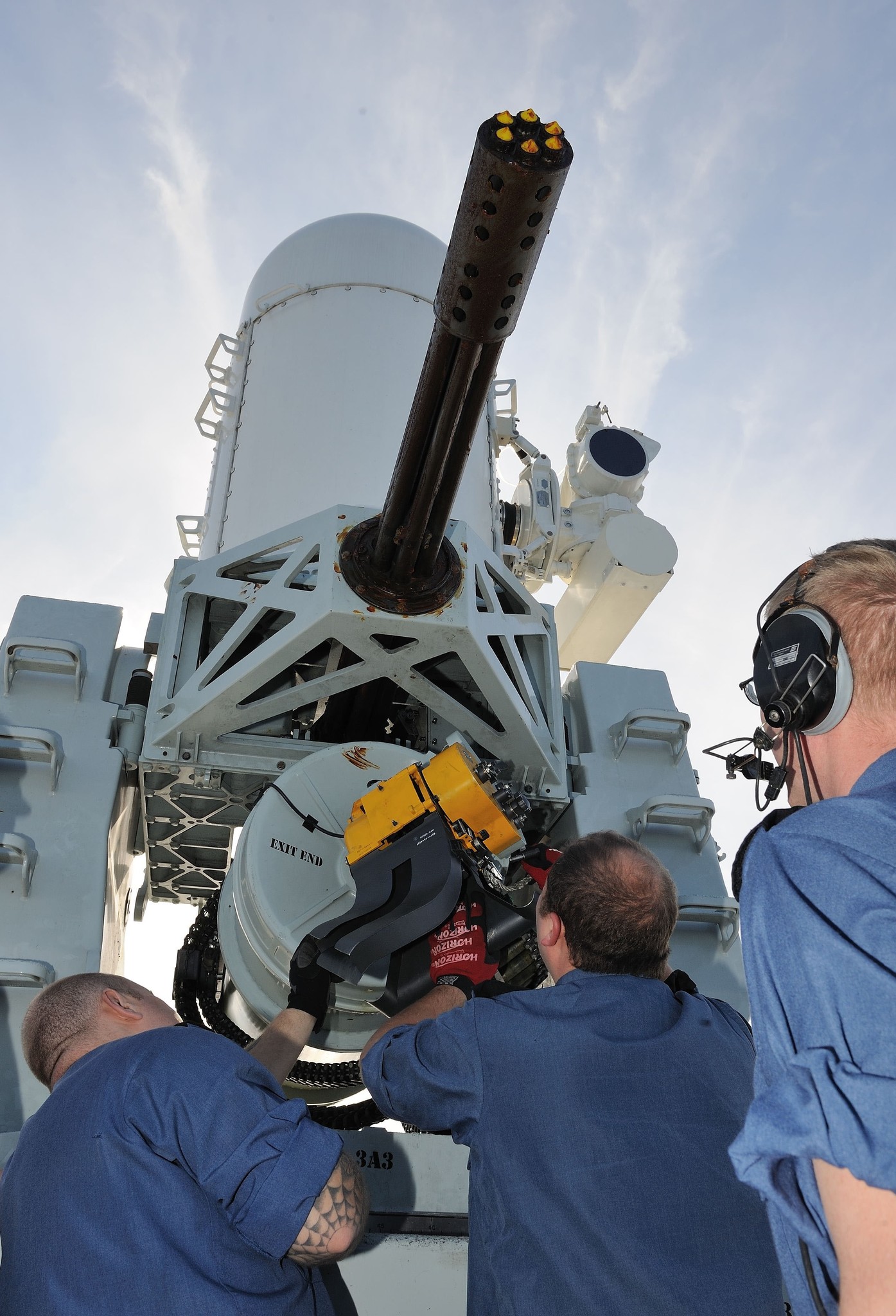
[522,843,564,891]
[427,889,503,1001]
[286,933,347,1035]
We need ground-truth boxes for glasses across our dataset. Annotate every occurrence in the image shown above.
[739,676,761,706]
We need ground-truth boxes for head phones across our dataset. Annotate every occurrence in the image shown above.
[752,539,896,738]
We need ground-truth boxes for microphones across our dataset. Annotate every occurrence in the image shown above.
[702,722,790,812]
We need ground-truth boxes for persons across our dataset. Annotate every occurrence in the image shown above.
[0,974,368,1316]
[359,828,789,1316]
[722,541,895,1316]
